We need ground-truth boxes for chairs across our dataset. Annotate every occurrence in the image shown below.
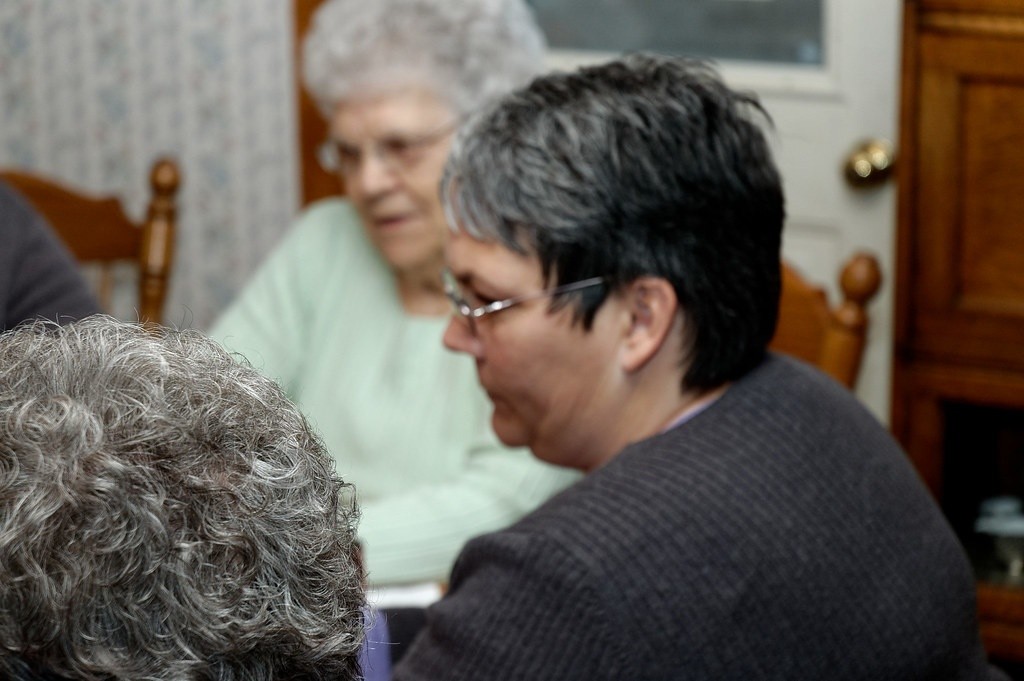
[765,254,882,392]
[0,158,180,324]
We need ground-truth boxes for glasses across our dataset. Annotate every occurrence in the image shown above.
[424,274,609,335]
[317,107,473,175]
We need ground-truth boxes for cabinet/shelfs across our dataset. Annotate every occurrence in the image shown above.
[891,0,1023,659]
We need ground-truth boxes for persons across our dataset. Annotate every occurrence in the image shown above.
[192,2,591,679]
[374,53,995,681]
